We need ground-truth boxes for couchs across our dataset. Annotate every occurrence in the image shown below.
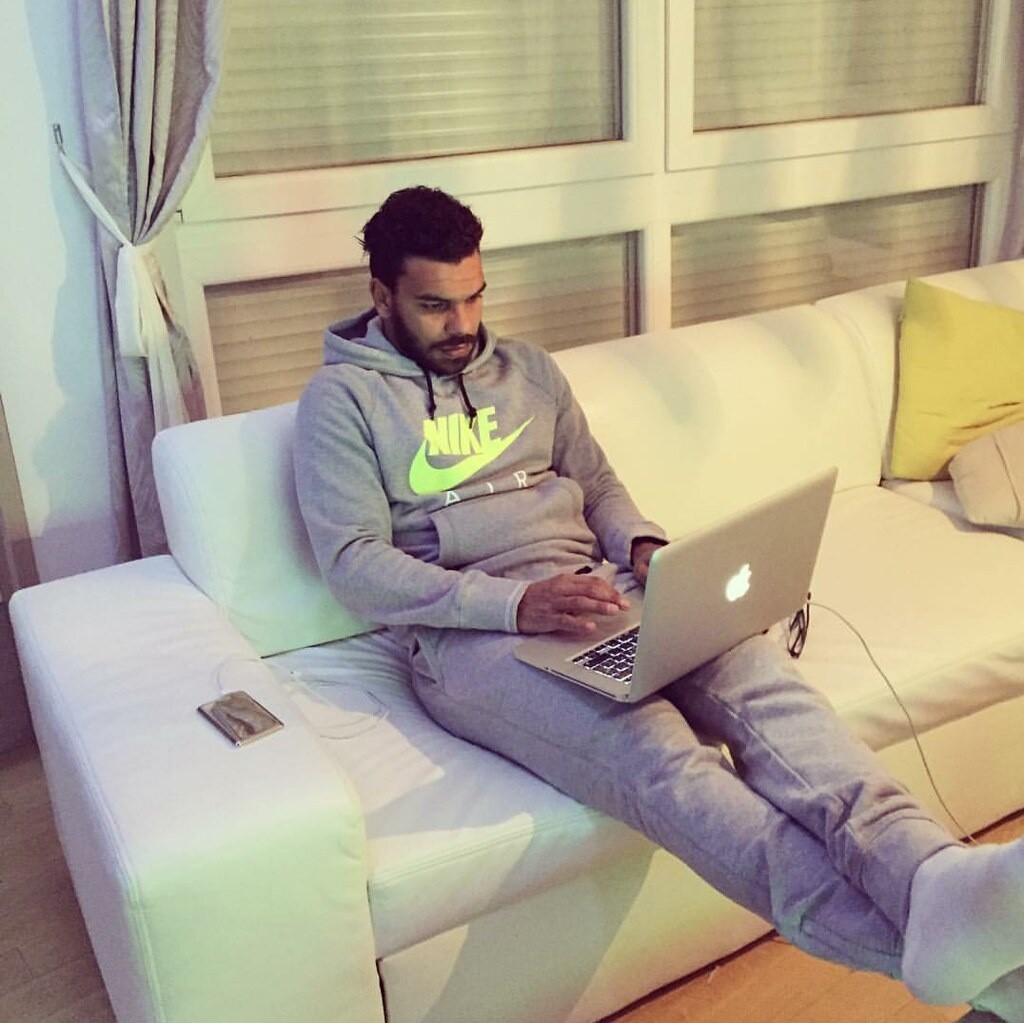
[6,251,1024,1023]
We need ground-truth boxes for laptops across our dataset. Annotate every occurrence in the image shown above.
[511,468,839,703]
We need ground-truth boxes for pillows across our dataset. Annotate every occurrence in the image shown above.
[949,413,1023,535]
[881,277,1022,481]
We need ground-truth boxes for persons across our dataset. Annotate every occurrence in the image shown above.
[292,182,1024,1012]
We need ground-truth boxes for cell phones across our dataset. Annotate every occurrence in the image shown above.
[198,690,284,747]
